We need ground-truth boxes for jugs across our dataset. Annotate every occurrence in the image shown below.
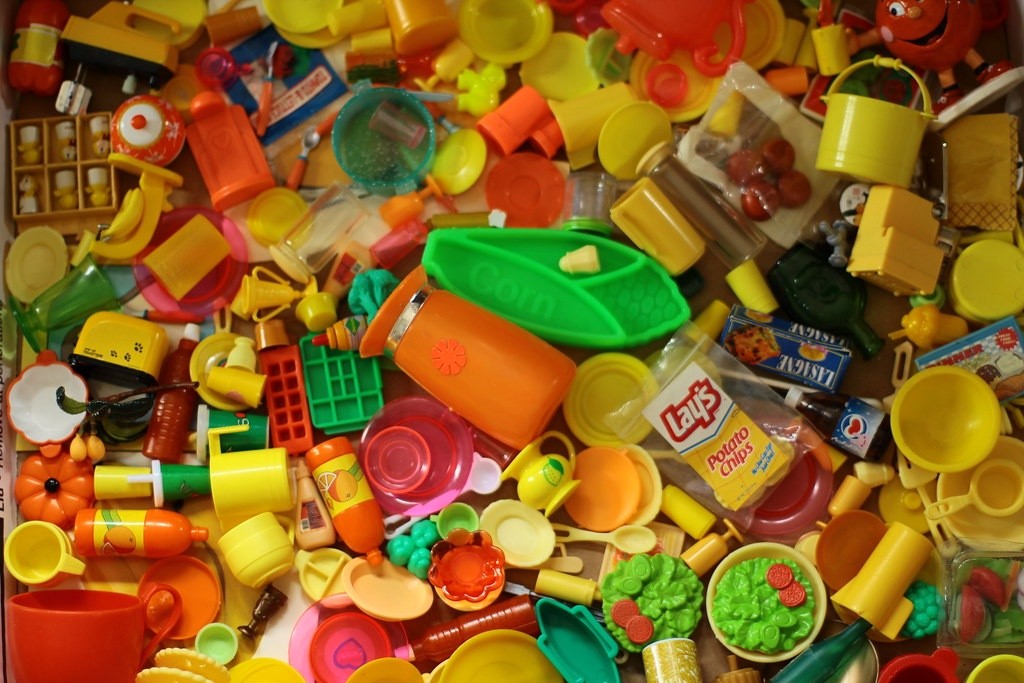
[359,266,576,450]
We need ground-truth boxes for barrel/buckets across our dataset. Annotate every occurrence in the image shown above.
[816,61,937,188]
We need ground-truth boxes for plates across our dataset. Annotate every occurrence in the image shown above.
[597,101,673,181]
[138,552,220,640]
[357,399,476,519]
[133,209,249,321]
[454,2,557,63]
[567,353,659,447]
[343,556,435,621]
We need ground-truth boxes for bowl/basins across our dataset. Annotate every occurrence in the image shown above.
[362,429,430,497]
[892,364,1005,472]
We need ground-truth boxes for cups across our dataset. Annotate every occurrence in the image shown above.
[0,1,1024,683]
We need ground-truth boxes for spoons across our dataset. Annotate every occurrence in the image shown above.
[286,125,323,189]
[551,521,658,555]
[381,451,502,540]
[255,39,278,134]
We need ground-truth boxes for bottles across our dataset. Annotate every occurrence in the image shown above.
[778,387,892,464]
[769,618,873,682]
[770,242,887,359]
[395,595,539,663]
